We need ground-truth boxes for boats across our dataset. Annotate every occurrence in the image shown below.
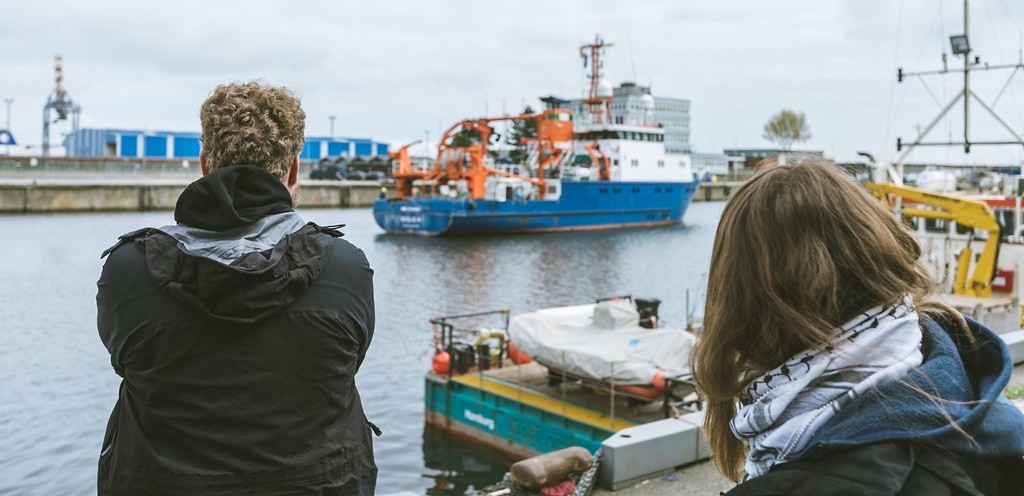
[852,0,1024,371]
[421,291,715,496]
[372,34,705,239]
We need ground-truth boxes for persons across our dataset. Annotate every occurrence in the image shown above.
[95,83,379,496]
[692,160,1024,496]
